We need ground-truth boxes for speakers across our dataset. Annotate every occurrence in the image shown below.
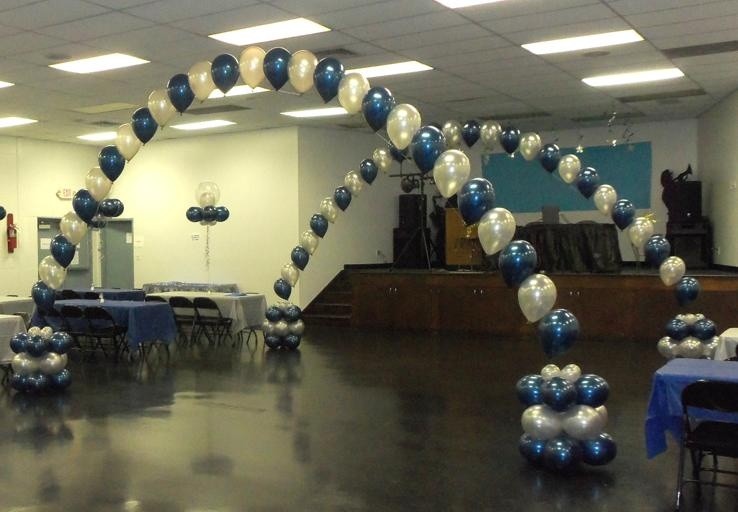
[392,228,431,267]
[673,181,702,217]
[398,193,428,227]
[668,233,713,272]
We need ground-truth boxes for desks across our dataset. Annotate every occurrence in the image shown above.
[512,223,625,272]
[714,328,738,361]
[645,357,738,511]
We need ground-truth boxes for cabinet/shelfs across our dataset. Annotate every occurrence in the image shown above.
[444,208,484,266]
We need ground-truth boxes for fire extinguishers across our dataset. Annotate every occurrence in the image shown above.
[7,223,20,248]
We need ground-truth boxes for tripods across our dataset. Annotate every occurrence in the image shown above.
[390,177,443,271]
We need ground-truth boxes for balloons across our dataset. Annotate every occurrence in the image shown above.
[0,206,6,220]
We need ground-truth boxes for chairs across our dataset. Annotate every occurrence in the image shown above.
[0,281,266,386]
[680,380,738,499]
[631,241,650,273]
